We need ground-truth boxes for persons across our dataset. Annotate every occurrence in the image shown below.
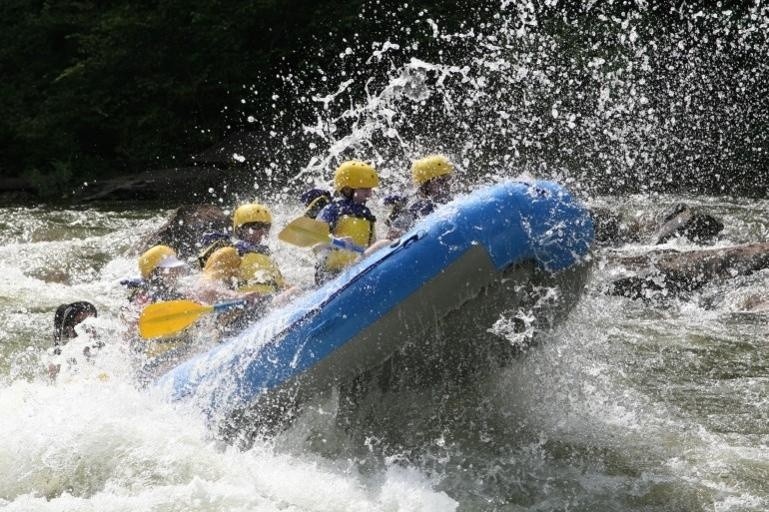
[48,301,98,380]
[386,155,455,241]
[305,161,393,289]
[194,205,299,341]
[128,245,199,386]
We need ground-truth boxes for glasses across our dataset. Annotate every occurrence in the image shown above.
[251,223,269,229]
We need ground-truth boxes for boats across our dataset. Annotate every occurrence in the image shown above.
[134,175,595,481]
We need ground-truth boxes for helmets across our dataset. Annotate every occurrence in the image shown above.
[233,205,271,229]
[333,162,378,193]
[412,156,451,183]
[139,246,176,277]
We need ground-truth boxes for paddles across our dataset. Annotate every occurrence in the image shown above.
[138,290,274,343]
[279,216,371,258]
